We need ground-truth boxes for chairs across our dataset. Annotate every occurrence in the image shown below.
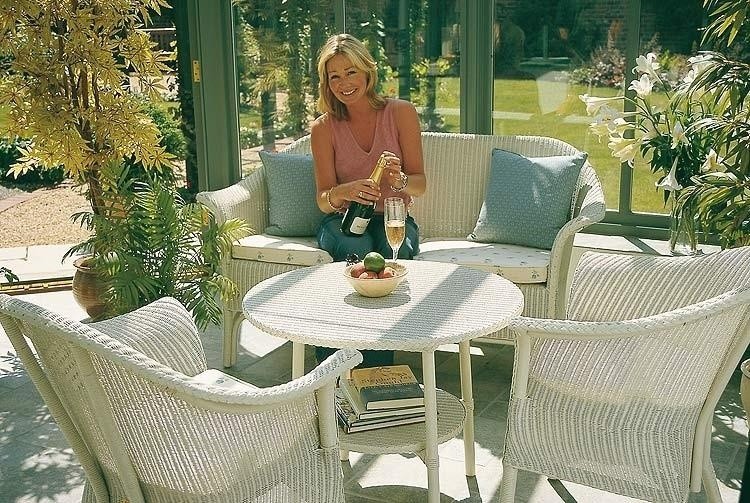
[0,291,363,503]
[501,246,750,501]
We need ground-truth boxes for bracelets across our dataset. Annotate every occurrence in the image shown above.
[388,171,409,192]
[325,185,347,211]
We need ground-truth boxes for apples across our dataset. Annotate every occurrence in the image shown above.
[350,251,397,280]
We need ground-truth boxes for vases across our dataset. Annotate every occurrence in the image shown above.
[740,360,750,426]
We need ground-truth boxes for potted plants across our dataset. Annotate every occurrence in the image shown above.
[0,1,175,322]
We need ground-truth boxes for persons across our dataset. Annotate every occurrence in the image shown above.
[311,32,429,387]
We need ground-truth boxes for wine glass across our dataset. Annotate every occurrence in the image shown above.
[384,196,406,265]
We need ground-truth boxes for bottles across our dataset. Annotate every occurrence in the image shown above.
[340,153,390,238]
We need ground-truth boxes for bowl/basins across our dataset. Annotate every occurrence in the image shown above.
[342,260,411,297]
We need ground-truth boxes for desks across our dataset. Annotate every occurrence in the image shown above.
[241,259,525,502]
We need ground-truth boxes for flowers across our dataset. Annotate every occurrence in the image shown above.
[576,0,749,255]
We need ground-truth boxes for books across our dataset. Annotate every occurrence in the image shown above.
[333,363,441,435]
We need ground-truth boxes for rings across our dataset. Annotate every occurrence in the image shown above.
[358,191,364,197]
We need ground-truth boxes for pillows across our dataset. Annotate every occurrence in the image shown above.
[466,148,588,250]
[257,146,327,237]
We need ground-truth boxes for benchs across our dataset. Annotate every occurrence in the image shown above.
[194,128,606,370]
